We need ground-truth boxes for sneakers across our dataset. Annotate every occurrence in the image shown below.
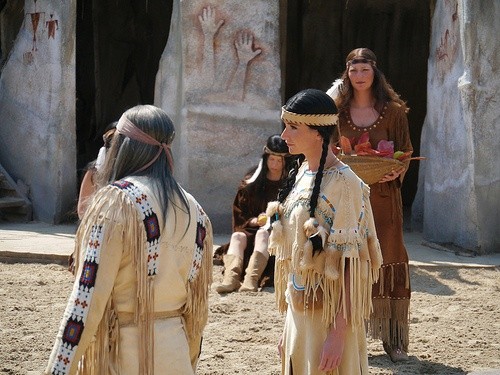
[383,341,408,363]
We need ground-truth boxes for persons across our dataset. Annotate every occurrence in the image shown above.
[42,104,213,375]
[77,120,118,220]
[213,135,296,294]
[267,90,383,375]
[325,48,413,362]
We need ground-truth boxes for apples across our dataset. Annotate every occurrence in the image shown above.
[257,213,267,225]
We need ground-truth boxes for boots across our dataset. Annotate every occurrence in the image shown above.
[238,251,267,292]
[216,254,244,292]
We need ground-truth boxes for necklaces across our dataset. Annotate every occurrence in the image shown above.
[277,156,340,217]
